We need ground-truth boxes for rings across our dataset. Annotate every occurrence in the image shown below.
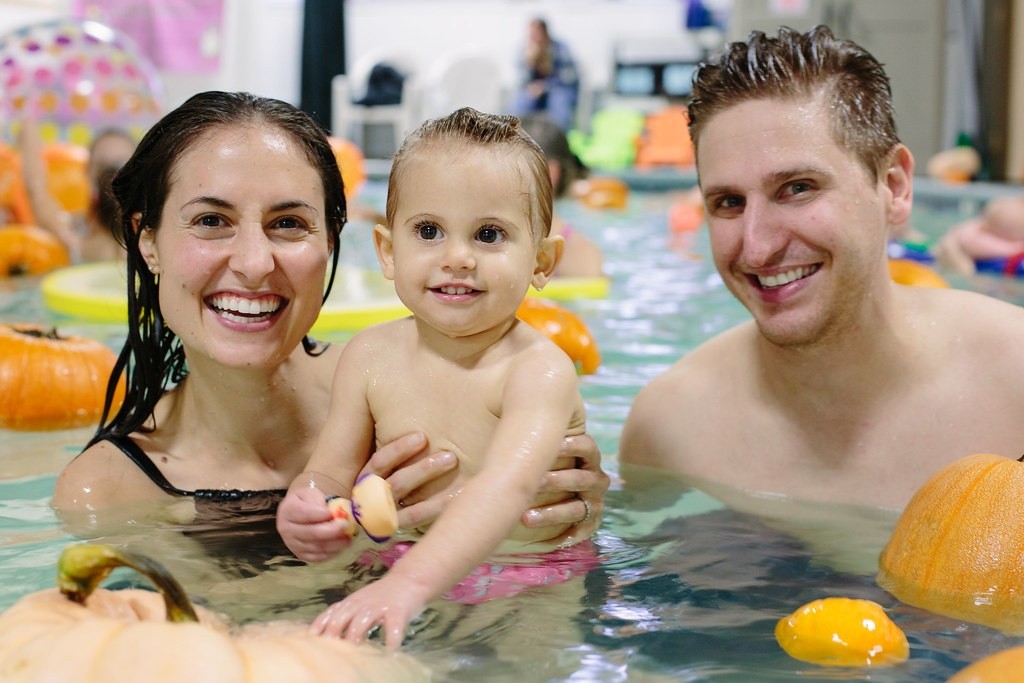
[568,495,594,523]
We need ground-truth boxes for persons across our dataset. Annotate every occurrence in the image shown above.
[19,17,1024,649]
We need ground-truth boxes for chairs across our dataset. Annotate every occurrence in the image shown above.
[560,101,697,168]
[329,53,422,154]
